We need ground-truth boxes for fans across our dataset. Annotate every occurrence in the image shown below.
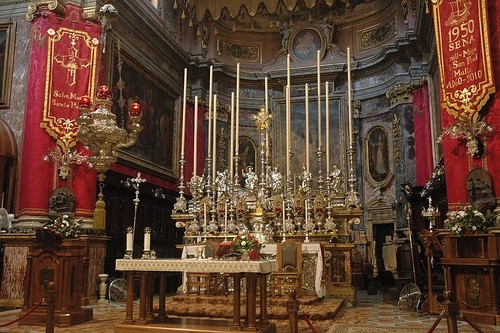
[107,278,128,303]
[398,283,423,312]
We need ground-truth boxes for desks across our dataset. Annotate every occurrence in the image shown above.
[115,259,279,333]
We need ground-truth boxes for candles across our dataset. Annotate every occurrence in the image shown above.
[79,86,142,116]
[180,46,353,237]
[143,227,151,252]
[126,226,133,251]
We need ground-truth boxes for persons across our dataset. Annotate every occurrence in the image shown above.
[215,164,344,202]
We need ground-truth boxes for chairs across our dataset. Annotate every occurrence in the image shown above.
[186,241,219,297]
[269,239,302,300]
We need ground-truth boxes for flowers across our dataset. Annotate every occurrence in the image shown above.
[441,201,493,247]
[227,232,266,255]
[45,214,84,239]
